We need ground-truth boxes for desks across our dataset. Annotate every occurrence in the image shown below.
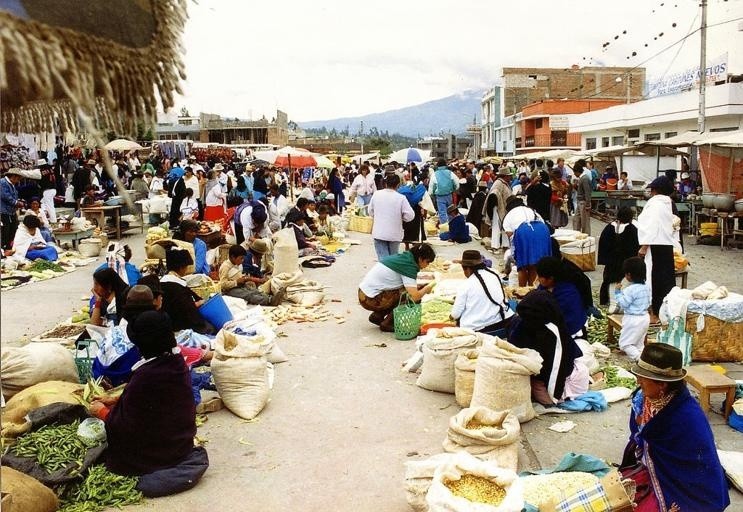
[79,202,121,241]
[104,203,144,238]
[52,224,96,248]
[590,190,743,253]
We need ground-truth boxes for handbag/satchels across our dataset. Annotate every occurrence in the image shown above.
[393,290,422,341]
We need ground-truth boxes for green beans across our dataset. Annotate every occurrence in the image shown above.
[1,418,143,512]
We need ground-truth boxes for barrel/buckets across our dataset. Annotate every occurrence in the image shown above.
[701,223,718,236]
[606,178,618,190]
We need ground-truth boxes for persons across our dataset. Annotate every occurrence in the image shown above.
[449,250,594,406]
[368,174,415,260]
[2,145,359,275]
[638,175,676,325]
[615,256,652,372]
[615,340,732,510]
[358,243,435,332]
[666,158,696,226]
[503,195,552,287]
[597,205,643,304]
[86,309,198,477]
[349,157,633,255]
[219,245,284,306]
[90,241,220,390]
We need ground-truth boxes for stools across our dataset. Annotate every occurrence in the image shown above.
[607,312,624,341]
[681,363,736,418]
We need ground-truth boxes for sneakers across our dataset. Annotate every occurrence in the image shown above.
[368,313,394,332]
[271,287,285,306]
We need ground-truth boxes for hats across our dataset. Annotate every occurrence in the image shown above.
[126,310,179,350]
[126,274,165,306]
[496,166,514,176]
[250,238,270,255]
[79,154,254,175]
[631,343,688,383]
[551,169,563,178]
[456,249,483,267]
[538,169,551,183]
[2,158,48,177]
